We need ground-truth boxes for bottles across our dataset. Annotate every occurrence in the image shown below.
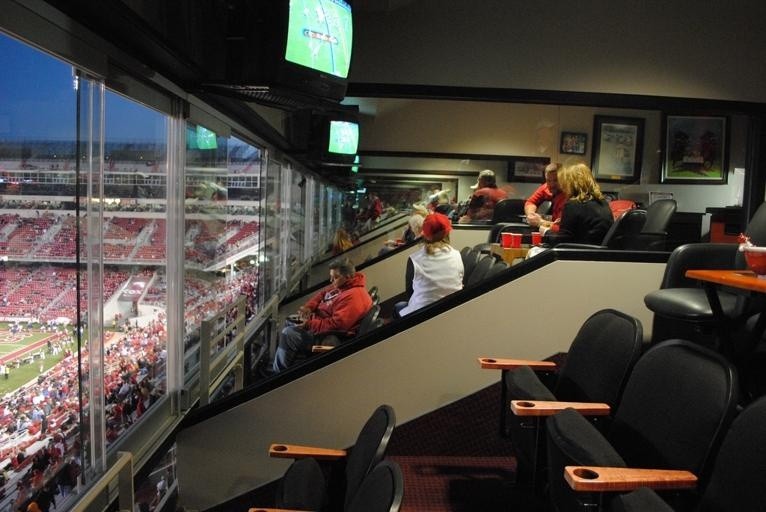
[429,189,451,203]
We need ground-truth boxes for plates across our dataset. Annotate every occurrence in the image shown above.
[284,313,305,323]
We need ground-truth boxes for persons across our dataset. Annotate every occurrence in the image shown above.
[459,169,508,224]
[272,258,372,376]
[1,188,275,511]
[392,213,464,320]
[354,192,383,234]
[378,204,430,256]
[524,157,614,258]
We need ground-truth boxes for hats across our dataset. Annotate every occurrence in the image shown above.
[423,213,451,242]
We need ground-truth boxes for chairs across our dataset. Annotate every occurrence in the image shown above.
[248,401,403,512]
[643,196,765,350]
[323,194,679,252]
[284,285,387,356]
[475,304,765,511]
[395,245,508,320]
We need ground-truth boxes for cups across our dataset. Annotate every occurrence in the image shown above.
[512,234,523,248]
[531,232,540,245]
[501,232,513,247]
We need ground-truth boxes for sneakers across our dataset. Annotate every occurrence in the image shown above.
[259,365,275,377]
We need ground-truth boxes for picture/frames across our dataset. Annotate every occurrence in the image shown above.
[658,111,732,185]
[591,113,646,185]
[559,131,588,155]
[506,159,550,184]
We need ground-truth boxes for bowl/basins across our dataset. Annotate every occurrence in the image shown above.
[744,246,766,277]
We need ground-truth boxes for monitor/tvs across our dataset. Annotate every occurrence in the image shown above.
[224,1,355,102]
[312,116,361,164]
